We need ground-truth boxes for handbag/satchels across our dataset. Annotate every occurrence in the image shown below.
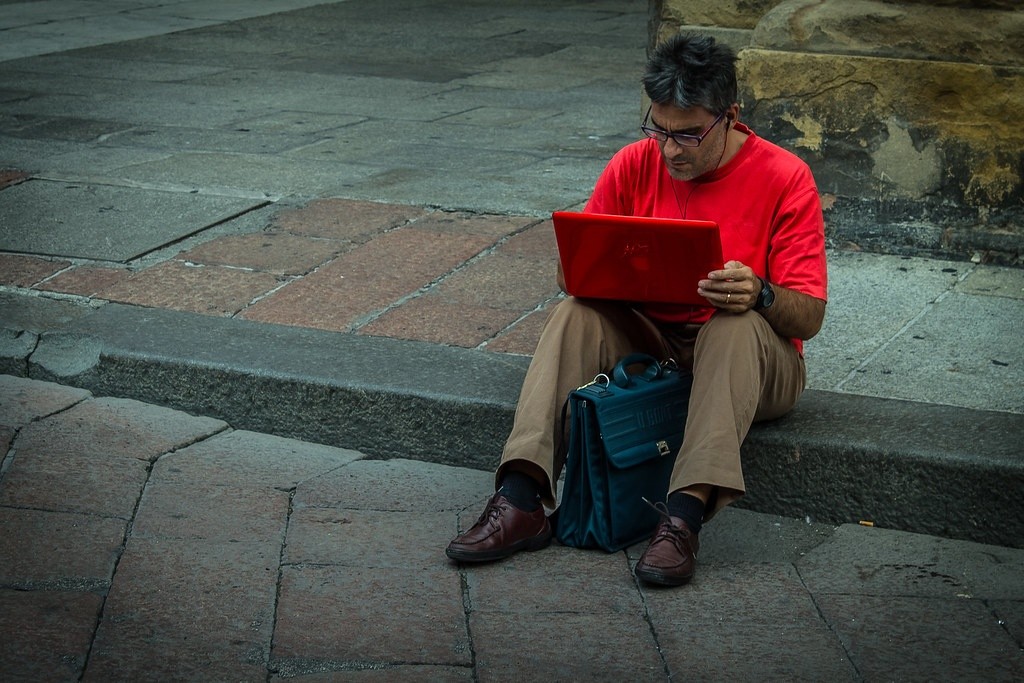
[547,352,694,554]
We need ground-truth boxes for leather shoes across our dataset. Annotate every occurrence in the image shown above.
[446,483,553,566]
[635,510,702,589]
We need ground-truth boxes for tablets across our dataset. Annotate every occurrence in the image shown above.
[552,212,723,308]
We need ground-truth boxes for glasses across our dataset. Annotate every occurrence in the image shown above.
[641,105,727,147]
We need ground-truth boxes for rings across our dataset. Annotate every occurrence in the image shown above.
[726,294,730,303]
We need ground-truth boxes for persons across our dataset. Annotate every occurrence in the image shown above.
[445,30,827,585]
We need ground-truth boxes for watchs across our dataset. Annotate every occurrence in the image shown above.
[754,276,775,311]
